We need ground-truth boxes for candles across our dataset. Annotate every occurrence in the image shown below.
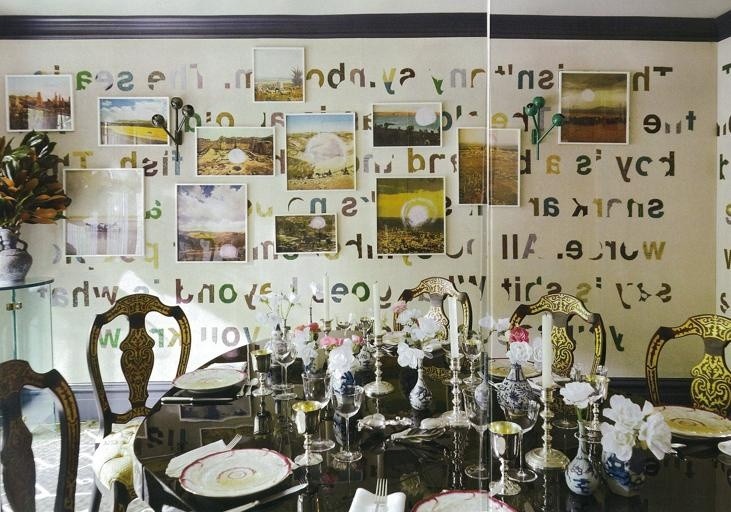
[373,280,382,334]
[541,309,553,386]
[323,269,330,320]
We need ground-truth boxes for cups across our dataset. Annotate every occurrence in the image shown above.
[293,401,324,466]
[250,349,272,397]
[488,421,522,496]
[254,398,293,438]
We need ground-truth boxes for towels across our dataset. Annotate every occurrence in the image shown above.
[347,487,407,512]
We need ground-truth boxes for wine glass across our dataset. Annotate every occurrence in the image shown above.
[331,385,365,463]
[272,340,299,402]
[503,399,541,483]
[268,331,297,391]
[461,388,491,480]
[301,372,336,453]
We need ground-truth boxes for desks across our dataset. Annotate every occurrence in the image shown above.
[0,277,54,429]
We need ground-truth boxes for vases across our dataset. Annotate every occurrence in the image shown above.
[331,405,363,449]
[496,363,648,497]
[409,368,435,410]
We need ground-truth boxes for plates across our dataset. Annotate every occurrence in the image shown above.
[408,250,731,489]
[488,358,542,379]
[172,368,248,394]
[176,447,293,499]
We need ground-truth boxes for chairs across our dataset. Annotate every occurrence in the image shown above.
[392,276,473,342]
[0,360,80,512]
[503,292,607,380]
[86,294,192,512]
[645,313,731,416]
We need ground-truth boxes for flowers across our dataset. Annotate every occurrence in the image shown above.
[256,283,447,396]
[505,326,672,461]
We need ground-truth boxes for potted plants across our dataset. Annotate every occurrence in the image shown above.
[0,125,73,283]
